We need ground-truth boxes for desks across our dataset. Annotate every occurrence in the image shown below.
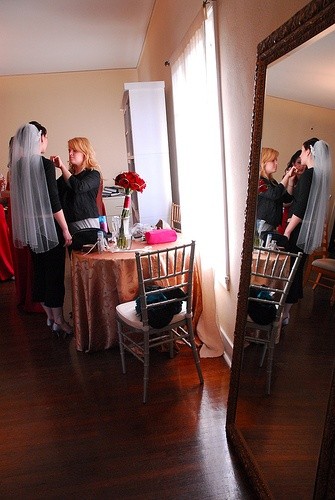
[62,234,203,353]
[250,253,293,343]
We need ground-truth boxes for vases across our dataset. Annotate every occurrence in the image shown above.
[118,217,132,249]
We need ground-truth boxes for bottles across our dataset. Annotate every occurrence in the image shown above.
[98,216,108,233]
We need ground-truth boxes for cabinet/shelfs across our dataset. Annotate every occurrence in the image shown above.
[121,81,174,225]
[102,193,134,230]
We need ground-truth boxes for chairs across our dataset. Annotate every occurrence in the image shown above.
[244,245,302,394]
[171,202,181,232]
[118,244,204,404]
[304,245,335,302]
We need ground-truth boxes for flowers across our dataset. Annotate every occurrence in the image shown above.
[113,172,146,216]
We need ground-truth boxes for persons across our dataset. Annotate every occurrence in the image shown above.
[50,137,103,320]
[7,120,75,338]
[252,137,331,326]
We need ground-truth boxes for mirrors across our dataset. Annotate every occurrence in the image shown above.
[226,1,335,500]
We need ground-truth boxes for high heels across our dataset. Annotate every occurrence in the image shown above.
[46,318,73,337]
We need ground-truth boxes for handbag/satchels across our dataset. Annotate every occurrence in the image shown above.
[135,284,186,329]
[248,283,277,325]
